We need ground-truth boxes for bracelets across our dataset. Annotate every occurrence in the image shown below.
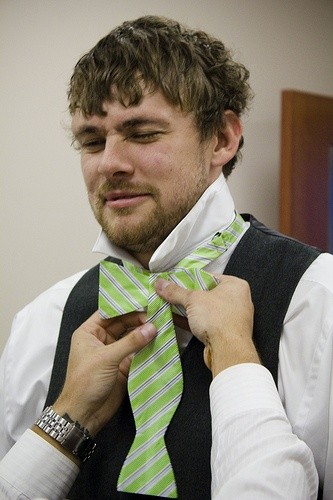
[35,406,96,463]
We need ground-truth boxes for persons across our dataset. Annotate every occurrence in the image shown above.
[1,15,333,500]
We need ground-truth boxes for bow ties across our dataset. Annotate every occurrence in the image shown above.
[99,209,250,498]
